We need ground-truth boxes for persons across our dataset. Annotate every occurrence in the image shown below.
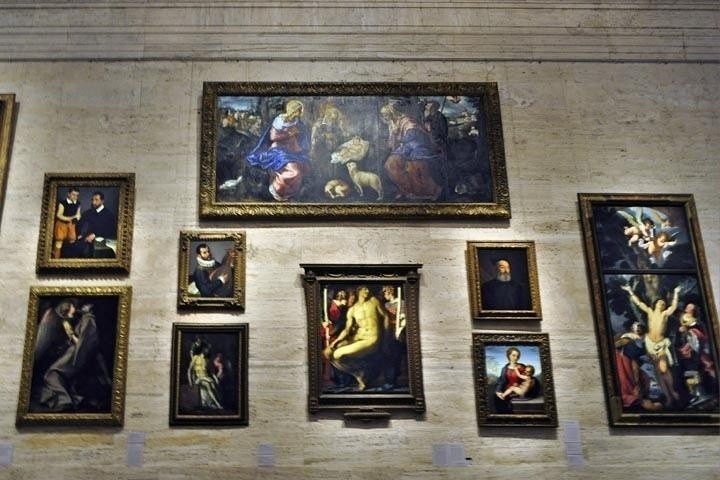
[53,188,115,259]
[186,336,224,410]
[36,296,97,408]
[623,205,678,264]
[194,243,235,298]
[494,347,540,413]
[614,284,711,411]
[481,256,529,309]
[322,284,407,390]
[268,98,448,200]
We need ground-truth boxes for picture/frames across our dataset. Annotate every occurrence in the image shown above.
[0,92,18,231]
[468,331,561,432]
[298,260,429,417]
[34,168,138,275]
[15,284,135,431]
[175,227,249,313]
[197,77,514,224]
[573,190,720,431]
[464,238,544,322]
[168,320,251,426]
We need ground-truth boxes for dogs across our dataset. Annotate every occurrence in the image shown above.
[346,161,385,201]
[324,179,352,200]
